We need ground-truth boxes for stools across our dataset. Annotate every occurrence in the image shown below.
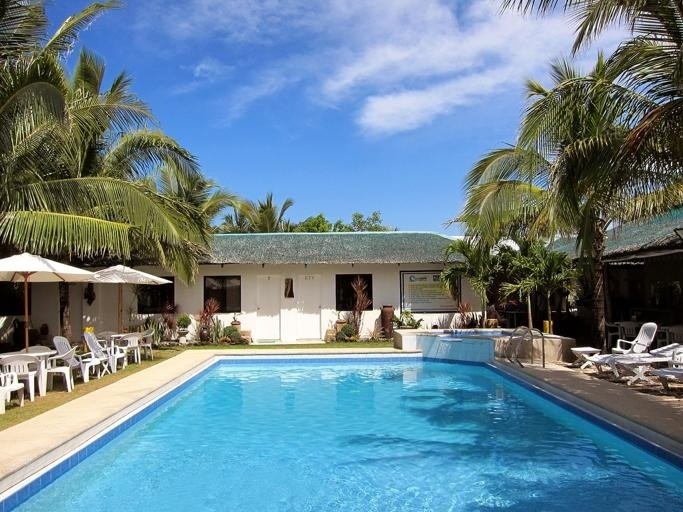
[570,346,601,367]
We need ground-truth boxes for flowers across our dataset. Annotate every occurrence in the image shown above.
[197,296,219,327]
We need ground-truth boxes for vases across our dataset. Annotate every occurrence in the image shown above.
[380,303,393,341]
[199,326,209,346]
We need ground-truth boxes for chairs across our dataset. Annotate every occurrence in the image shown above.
[579,321,683,395]
[0,326,155,416]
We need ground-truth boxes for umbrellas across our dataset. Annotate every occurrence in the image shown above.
[89,264,174,334]
[0,250,102,355]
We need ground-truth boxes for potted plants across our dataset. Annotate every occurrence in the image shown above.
[176,314,191,336]
[231,312,242,333]
